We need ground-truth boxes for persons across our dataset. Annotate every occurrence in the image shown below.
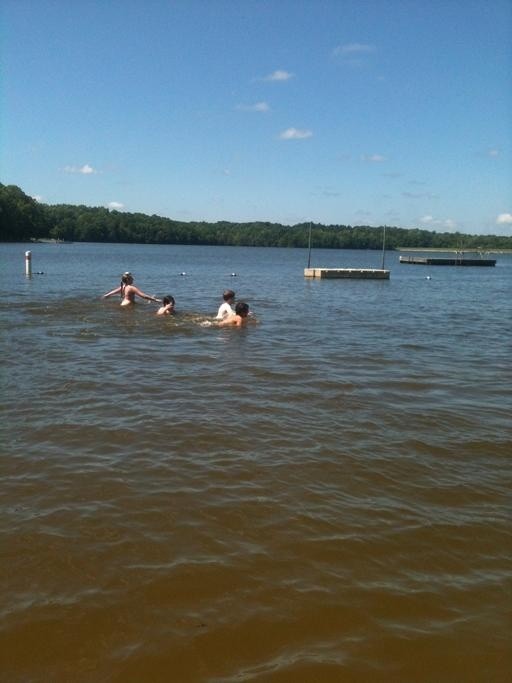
[209,302,250,326]
[216,289,236,318]
[156,295,176,315]
[100,273,163,306]
